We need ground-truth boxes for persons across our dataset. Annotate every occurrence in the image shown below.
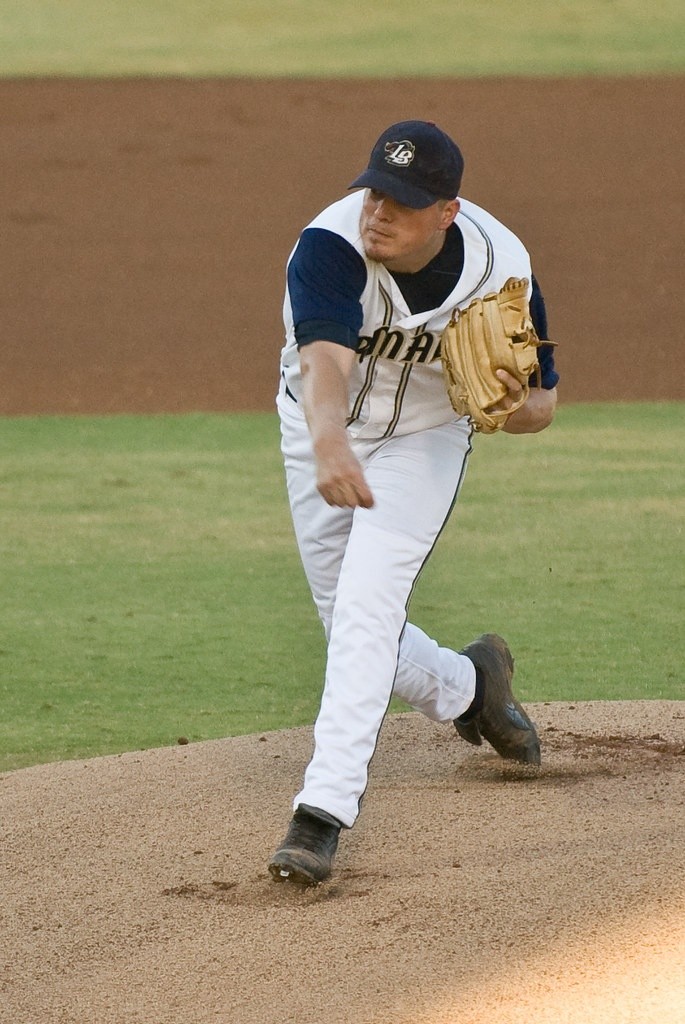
[267,119,558,887]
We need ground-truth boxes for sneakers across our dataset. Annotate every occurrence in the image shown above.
[457,633,542,763]
[268,803,342,887]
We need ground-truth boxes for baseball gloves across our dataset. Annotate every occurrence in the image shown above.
[439,276,558,434]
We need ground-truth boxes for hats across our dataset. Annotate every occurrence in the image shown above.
[347,119,465,208]
